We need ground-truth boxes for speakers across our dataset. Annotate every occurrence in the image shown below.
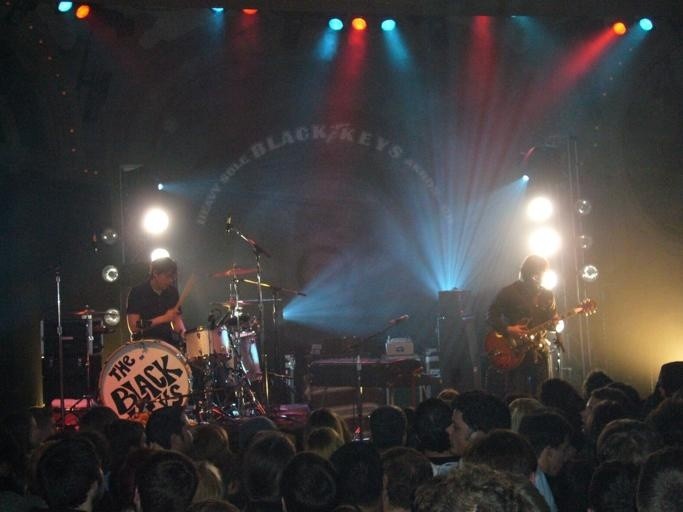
[437,287,470,316]
[436,314,481,391]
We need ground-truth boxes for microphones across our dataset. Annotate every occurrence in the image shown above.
[389,314,409,324]
[224,215,232,233]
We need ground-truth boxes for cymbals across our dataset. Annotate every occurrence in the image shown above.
[216,268,257,276]
[70,308,106,317]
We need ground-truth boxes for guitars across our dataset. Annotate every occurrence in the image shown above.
[484,299,597,368]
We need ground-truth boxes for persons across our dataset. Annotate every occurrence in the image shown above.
[1,356,683,512]
[488,253,562,397]
[126,257,186,350]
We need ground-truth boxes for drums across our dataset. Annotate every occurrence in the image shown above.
[220,331,264,386]
[99,339,193,427]
[185,325,232,361]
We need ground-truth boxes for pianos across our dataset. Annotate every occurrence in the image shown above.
[311,355,421,388]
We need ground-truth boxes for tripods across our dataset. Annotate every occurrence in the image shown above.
[68,314,102,411]
[195,228,308,422]
